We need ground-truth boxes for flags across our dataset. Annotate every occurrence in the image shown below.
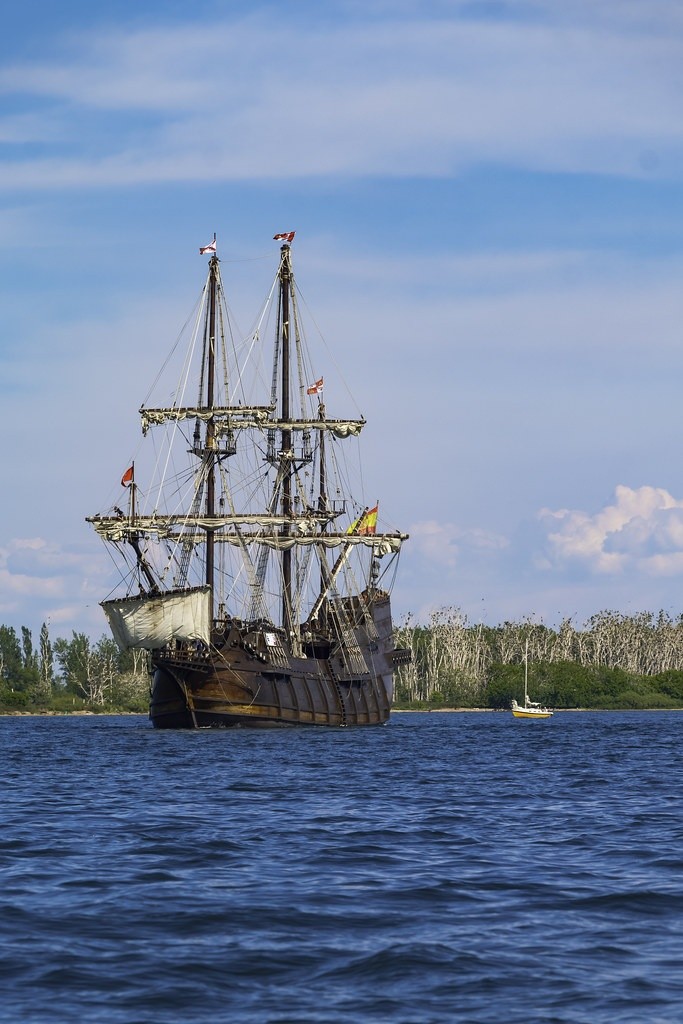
[306,377,322,395]
[344,505,378,534]
[121,466,133,487]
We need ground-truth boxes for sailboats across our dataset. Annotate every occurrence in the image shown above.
[86,231,411,730]
[512,639,553,719]
